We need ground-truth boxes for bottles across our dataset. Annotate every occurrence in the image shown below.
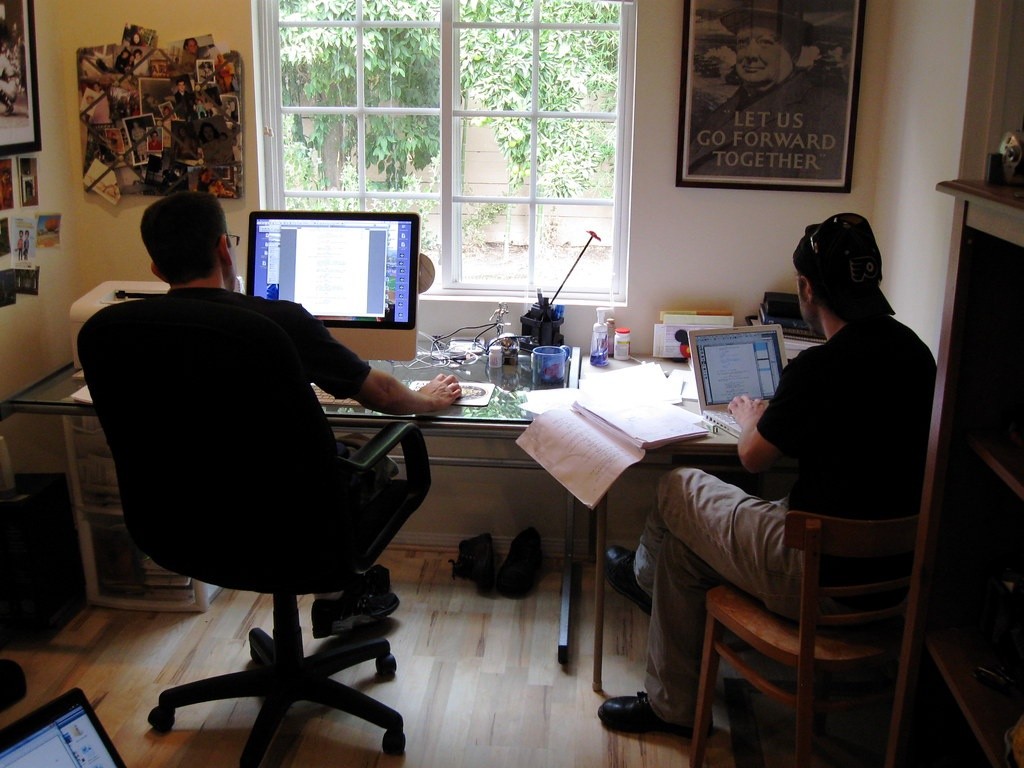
[614,327,631,361]
[489,346,503,368]
[605,319,616,357]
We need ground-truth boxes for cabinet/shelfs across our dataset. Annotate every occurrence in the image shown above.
[889,175,1024,768]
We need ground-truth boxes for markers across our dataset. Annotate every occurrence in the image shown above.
[543,297,549,322]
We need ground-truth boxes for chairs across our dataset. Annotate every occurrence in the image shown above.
[76,301,433,768]
[689,508,922,768]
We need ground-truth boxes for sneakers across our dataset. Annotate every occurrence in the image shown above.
[312,565,399,639]
[448,533,493,597]
[498,527,542,598]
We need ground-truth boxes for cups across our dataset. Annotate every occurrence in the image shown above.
[533,345,571,390]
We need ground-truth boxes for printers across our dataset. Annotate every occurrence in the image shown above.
[69,280,171,369]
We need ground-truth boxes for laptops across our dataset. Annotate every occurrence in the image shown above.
[0,687,127,768]
[687,324,788,438]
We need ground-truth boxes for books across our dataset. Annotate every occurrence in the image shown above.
[515,388,709,510]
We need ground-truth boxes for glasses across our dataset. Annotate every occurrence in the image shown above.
[226,234,240,248]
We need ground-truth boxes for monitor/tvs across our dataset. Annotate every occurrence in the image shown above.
[247,211,421,362]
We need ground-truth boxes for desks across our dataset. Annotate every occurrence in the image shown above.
[0,340,745,668]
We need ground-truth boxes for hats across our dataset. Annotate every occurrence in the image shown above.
[794,213,896,322]
[719,0,818,46]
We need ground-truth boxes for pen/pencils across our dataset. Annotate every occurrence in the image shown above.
[537,288,543,309]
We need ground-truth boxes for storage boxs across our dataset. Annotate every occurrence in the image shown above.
[69,280,246,372]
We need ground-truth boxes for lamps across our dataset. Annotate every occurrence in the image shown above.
[674,0,867,194]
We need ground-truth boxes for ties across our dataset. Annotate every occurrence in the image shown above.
[727,87,755,125]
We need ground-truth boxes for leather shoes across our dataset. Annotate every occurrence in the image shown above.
[598,692,714,739]
[606,545,654,619]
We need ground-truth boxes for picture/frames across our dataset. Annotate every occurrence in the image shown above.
[0,0,43,158]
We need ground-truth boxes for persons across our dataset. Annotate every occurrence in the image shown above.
[140,192,461,640]
[686,0,848,185]
[77,23,242,201]
[598,212,936,737]
